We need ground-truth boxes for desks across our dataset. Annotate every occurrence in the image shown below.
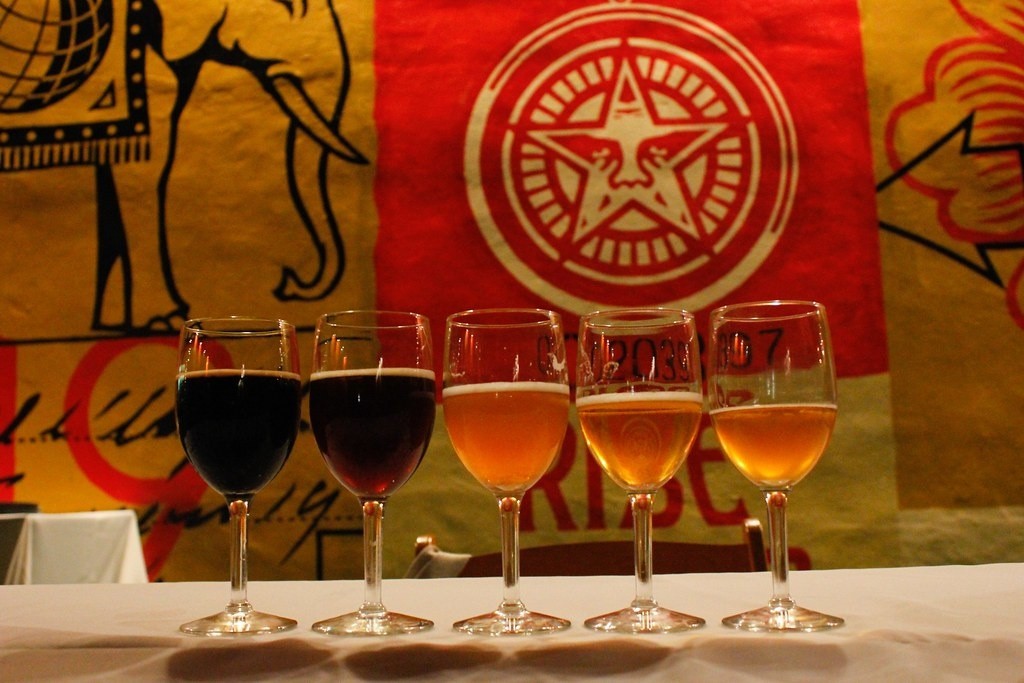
[0,563,1024,683]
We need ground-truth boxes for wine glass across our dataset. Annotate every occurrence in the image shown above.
[176,318,301,637]
[707,299,845,633]
[441,310,570,633]
[310,312,435,633]
[574,307,704,634]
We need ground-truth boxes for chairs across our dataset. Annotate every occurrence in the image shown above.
[4,510,149,585]
[411,520,768,580]
